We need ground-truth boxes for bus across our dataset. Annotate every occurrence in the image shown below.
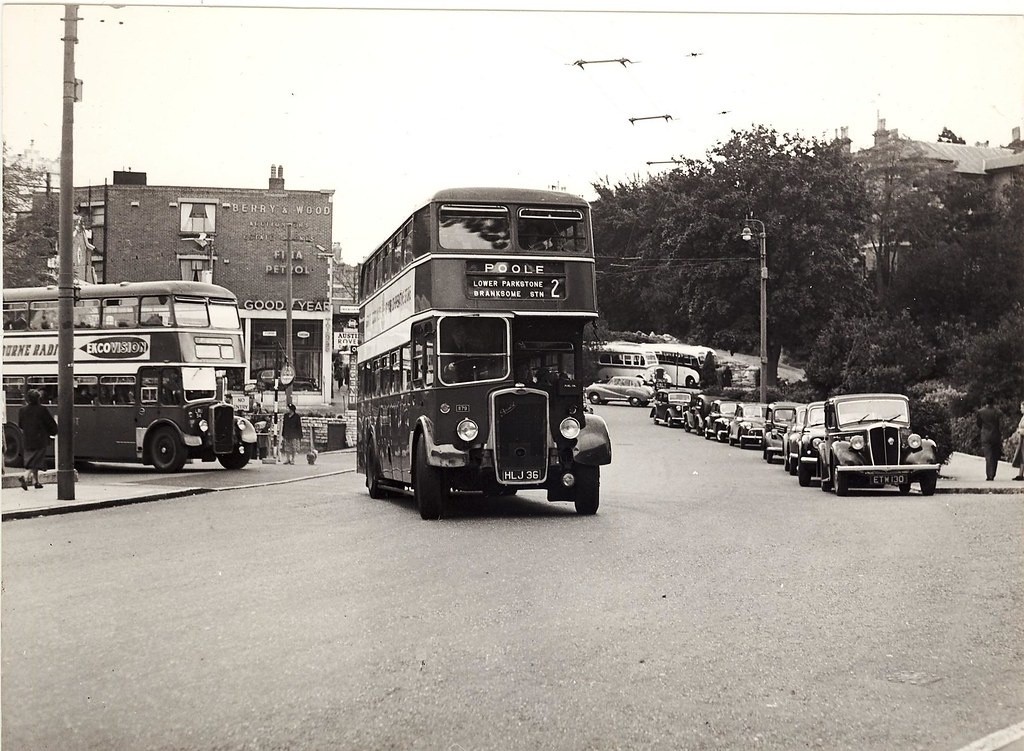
[587,346,659,385]
[357,187,611,520]
[642,343,719,390]
[1,280,257,474]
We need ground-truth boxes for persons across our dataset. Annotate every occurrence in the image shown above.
[18,389,58,491]
[249,402,271,459]
[224,393,233,405]
[79,385,132,405]
[281,404,302,465]
[976,395,1005,481]
[1012,401,1024,481]
[441,323,488,383]
[524,227,570,252]
[334,359,349,391]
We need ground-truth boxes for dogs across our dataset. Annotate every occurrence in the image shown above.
[307,450,318,464]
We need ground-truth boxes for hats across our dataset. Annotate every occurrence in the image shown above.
[983,396,995,404]
[287,403,296,412]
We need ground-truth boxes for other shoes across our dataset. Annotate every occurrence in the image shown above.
[18,476,28,491]
[34,482,43,489]
[1012,476,1024,481]
[986,477,993,481]
[283,460,295,465]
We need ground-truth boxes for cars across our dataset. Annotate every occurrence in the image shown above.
[233,367,319,392]
[584,377,654,407]
[650,389,940,496]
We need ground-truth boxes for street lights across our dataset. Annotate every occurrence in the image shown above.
[741,215,768,404]
